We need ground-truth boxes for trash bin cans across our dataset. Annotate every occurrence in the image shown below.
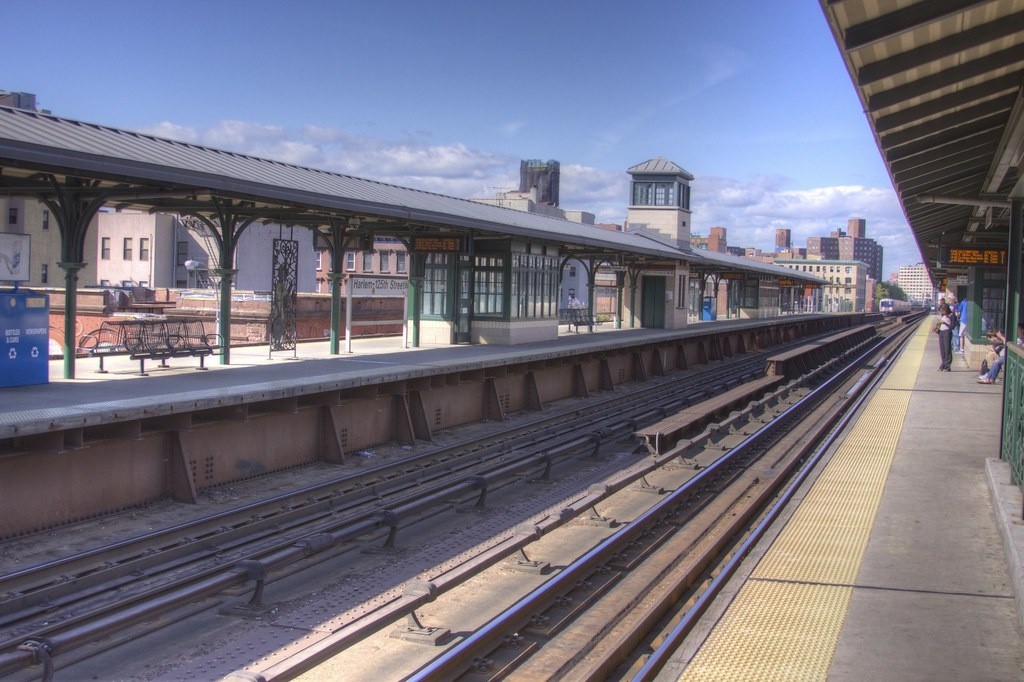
[0,286,54,389]
[702,296,717,321]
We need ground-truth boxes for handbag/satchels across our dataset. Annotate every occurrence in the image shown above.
[932,321,941,333]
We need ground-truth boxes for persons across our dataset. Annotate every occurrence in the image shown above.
[979,328,1003,376]
[936,303,956,371]
[977,323,1024,384]
[954,299,967,352]
[939,298,950,312]
[950,302,960,351]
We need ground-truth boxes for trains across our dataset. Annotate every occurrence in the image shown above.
[878,299,911,316]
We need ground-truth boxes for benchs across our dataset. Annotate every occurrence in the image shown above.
[76,317,228,377]
[558,307,600,334]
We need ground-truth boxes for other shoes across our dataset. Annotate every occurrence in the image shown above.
[937,367,951,372]
[960,349,964,352]
[977,379,993,384]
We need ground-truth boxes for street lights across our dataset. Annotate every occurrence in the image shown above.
[186,260,222,346]
